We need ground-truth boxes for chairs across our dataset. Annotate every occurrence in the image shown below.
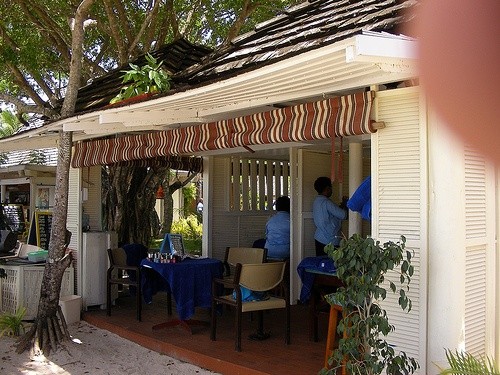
[212,246,291,351]
[106,248,144,321]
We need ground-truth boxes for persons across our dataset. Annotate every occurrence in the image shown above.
[412,1,500,169]
[312,177,348,257]
[265,196,290,258]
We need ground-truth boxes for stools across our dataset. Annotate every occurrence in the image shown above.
[324,303,363,375]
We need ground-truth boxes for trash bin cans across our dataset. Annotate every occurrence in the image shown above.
[53,295,81,325]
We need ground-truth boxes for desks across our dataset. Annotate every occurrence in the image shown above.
[0,265,74,321]
[141,256,225,336]
[297,256,358,344]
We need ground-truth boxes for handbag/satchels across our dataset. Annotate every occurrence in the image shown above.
[232,286,273,302]
